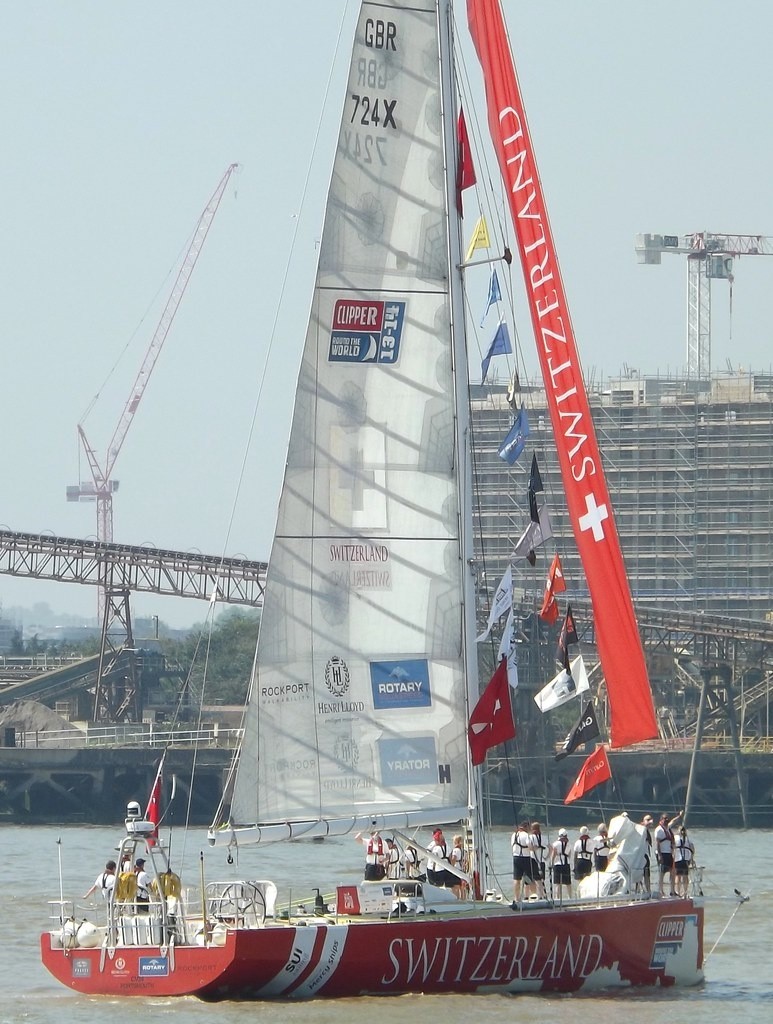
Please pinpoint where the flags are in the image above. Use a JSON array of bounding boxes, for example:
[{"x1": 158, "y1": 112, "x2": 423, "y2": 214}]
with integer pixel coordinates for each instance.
[
  {"x1": 556, "y1": 604, "x2": 578, "y2": 675},
  {"x1": 540, "y1": 552, "x2": 567, "y2": 627},
  {"x1": 555, "y1": 701, "x2": 600, "y2": 761},
  {"x1": 498, "y1": 606, "x2": 519, "y2": 689},
  {"x1": 468, "y1": 655, "x2": 516, "y2": 766},
  {"x1": 534, "y1": 655, "x2": 590, "y2": 714},
  {"x1": 146, "y1": 772, "x2": 161, "y2": 854},
  {"x1": 473, "y1": 562, "x2": 514, "y2": 644},
  {"x1": 455, "y1": 105, "x2": 553, "y2": 556},
  {"x1": 565, "y1": 745, "x2": 611, "y2": 804}
]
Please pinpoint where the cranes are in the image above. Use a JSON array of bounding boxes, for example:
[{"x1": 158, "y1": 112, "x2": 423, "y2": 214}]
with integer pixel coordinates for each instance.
[
  {"x1": 67, "y1": 161, "x2": 240, "y2": 630},
  {"x1": 634, "y1": 233, "x2": 773, "y2": 382}
]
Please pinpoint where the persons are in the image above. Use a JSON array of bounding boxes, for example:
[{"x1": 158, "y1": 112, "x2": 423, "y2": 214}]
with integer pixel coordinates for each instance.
[
  {"x1": 427, "y1": 828, "x2": 452, "y2": 889},
  {"x1": 524, "y1": 823, "x2": 553, "y2": 900},
  {"x1": 573, "y1": 823, "x2": 616, "y2": 883},
  {"x1": 549, "y1": 828, "x2": 571, "y2": 899},
  {"x1": 635, "y1": 809, "x2": 695, "y2": 897},
  {"x1": 405, "y1": 838, "x2": 425, "y2": 880},
  {"x1": 134, "y1": 858, "x2": 153, "y2": 915},
  {"x1": 449, "y1": 835, "x2": 462, "y2": 900},
  {"x1": 510, "y1": 821, "x2": 538, "y2": 902},
  {"x1": 82, "y1": 860, "x2": 117, "y2": 917},
  {"x1": 355, "y1": 832, "x2": 390, "y2": 881},
  {"x1": 385, "y1": 838, "x2": 399, "y2": 880}
]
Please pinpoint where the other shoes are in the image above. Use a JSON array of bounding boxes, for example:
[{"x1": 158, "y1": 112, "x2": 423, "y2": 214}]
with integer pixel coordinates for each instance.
[{"x1": 669, "y1": 892, "x2": 678, "y2": 896}]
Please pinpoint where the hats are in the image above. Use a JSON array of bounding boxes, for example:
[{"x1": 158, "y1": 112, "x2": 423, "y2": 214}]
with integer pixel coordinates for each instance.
[
  {"x1": 558, "y1": 828, "x2": 568, "y2": 837},
  {"x1": 531, "y1": 822, "x2": 541, "y2": 829},
  {"x1": 580, "y1": 826, "x2": 589, "y2": 835},
  {"x1": 136, "y1": 859, "x2": 146, "y2": 866},
  {"x1": 598, "y1": 823, "x2": 607, "y2": 833},
  {"x1": 106, "y1": 861, "x2": 116, "y2": 869},
  {"x1": 385, "y1": 839, "x2": 393, "y2": 842},
  {"x1": 643, "y1": 815, "x2": 654, "y2": 824}
]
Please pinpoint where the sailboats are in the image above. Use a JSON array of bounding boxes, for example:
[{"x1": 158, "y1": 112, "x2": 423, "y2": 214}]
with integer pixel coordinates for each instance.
[{"x1": 41, "y1": 0, "x2": 710, "y2": 1003}]
